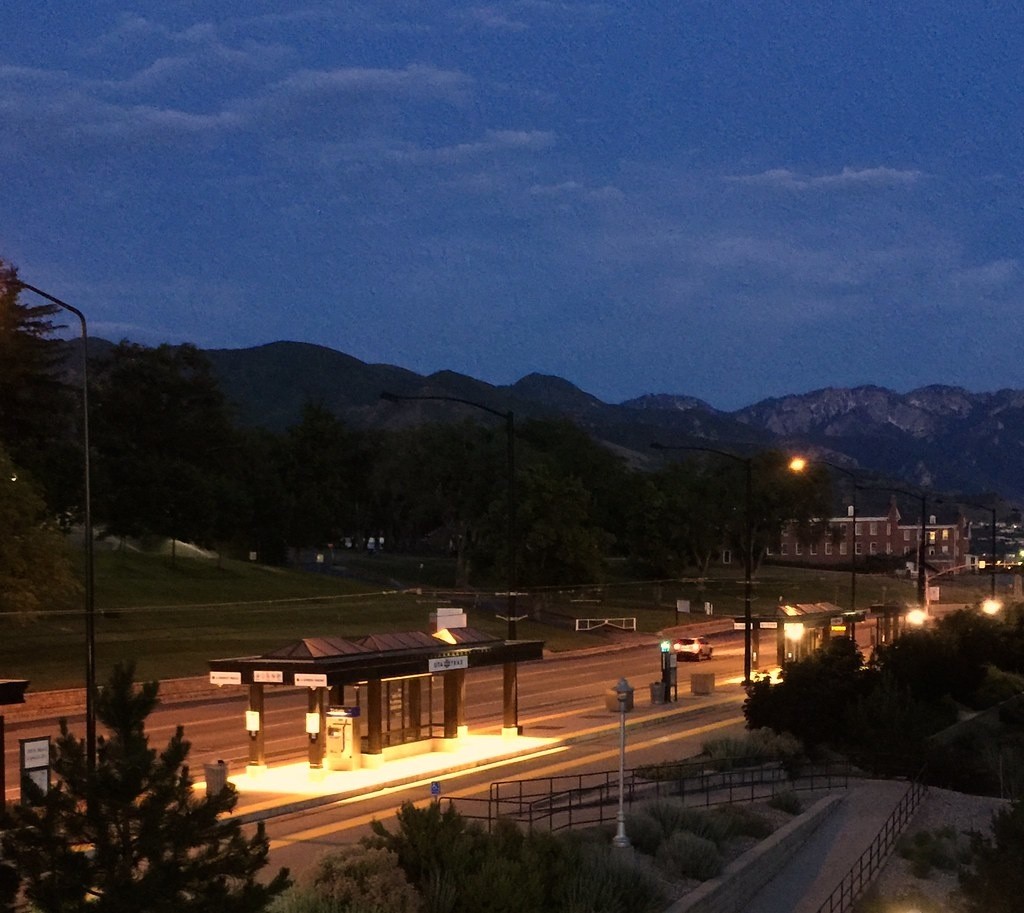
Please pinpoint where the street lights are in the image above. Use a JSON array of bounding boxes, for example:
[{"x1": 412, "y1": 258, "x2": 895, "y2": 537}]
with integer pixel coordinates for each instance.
[
  {"x1": 788, "y1": 457, "x2": 858, "y2": 645},
  {"x1": 854, "y1": 483, "x2": 927, "y2": 608},
  {"x1": 0, "y1": 278, "x2": 100, "y2": 827},
  {"x1": 379, "y1": 391, "x2": 518, "y2": 641},
  {"x1": 935, "y1": 496, "x2": 996, "y2": 601},
  {"x1": 609, "y1": 676, "x2": 636, "y2": 849},
  {"x1": 649, "y1": 440, "x2": 752, "y2": 684}
]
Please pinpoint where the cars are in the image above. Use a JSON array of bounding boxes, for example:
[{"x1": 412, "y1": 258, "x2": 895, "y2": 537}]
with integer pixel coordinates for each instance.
[{"x1": 673, "y1": 637, "x2": 714, "y2": 663}]
